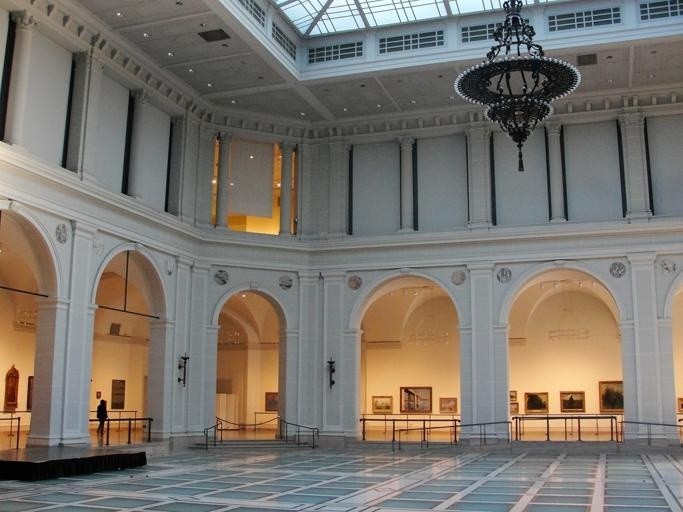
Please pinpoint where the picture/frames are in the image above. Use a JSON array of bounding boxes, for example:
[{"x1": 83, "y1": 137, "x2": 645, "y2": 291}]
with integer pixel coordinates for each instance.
[
  {"x1": 560, "y1": 390, "x2": 586, "y2": 412},
  {"x1": 372, "y1": 395, "x2": 394, "y2": 414},
  {"x1": 27, "y1": 375, "x2": 34, "y2": 411},
  {"x1": 678, "y1": 398, "x2": 683, "y2": 412},
  {"x1": 524, "y1": 392, "x2": 549, "y2": 413},
  {"x1": 400, "y1": 386, "x2": 432, "y2": 414},
  {"x1": 510, "y1": 402, "x2": 519, "y2": 414},
  {"x1": 598, "y1": 380, "x2": 624, "y2": 412},
  {"x1": 265, "y1": 392, "x2": 280, "y2": 412},
  {"x1": 440, "y1": 398, "x2": 457, "y2": 413},
  {"x1": 509, "y1": 391, "x2": 517, "y2": 401},
  {"x1": 5, "y1": 365, "x2": 19, "y2": 408}
]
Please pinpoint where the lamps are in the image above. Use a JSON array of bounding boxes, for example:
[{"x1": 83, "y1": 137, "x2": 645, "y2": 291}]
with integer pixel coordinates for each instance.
[
  {"x1": 327, "y1": 356, "x2": 335, "y2": 389},
  {"x1": 454, "y1": 0, "x2": 581, "y2": 170},
  {"x1": 177, "y1": 352, "x2": 189, "y2": 387}
]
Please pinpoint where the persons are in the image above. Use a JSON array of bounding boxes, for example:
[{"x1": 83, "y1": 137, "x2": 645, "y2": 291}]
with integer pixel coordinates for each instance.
[{"x1": 96, "y1": 400, "x2": 110, "y2": 435}]
